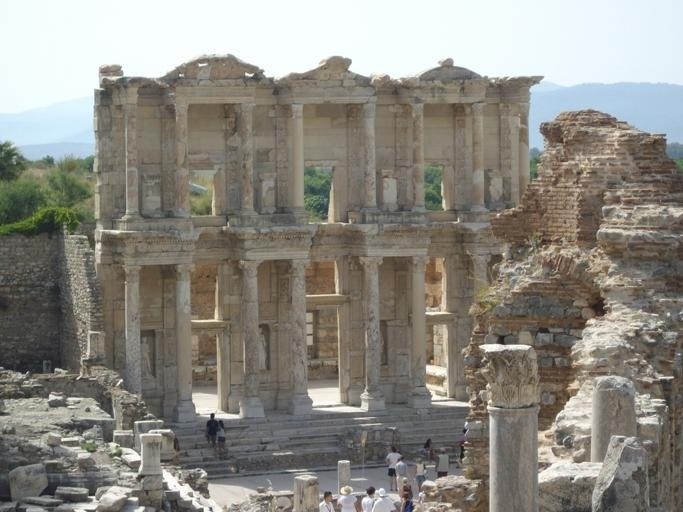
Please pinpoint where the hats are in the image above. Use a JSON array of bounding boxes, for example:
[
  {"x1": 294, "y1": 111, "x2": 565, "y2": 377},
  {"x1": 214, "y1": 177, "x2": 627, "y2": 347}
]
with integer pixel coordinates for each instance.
[
  {"x1": 379, "y1": 488, "x2": 386, "y2": 497},
  {"x1": 341, "y1": 486, "x2": 353, "y2": 495}
]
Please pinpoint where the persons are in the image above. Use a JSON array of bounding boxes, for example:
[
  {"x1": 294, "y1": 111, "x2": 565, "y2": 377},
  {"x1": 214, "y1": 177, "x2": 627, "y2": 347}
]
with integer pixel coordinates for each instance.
[
  {"x1": 360, "y1": 486, "x2": 375, "y2": 512},
  {"x1": 216, "y1": 419, "x2": 225, "y2": 453},
  {"x1": 318, "y1": 490, "x2": 335, "y2": 512},
  {"x1": 205, "y1": 413, "x2": 217, "y2": 449},
  {"x1": 336, "y1": 485, "x2": 362, "y2": 512},
  {"x1": 382, "y1": 437, "x2": 450, "y2": 511},
  {"x1": 456, "y1": 428, "x2": 467, "y2": 468},
  {"x1": 371, "y1": 488, "x2": 398, "y2": 511}
]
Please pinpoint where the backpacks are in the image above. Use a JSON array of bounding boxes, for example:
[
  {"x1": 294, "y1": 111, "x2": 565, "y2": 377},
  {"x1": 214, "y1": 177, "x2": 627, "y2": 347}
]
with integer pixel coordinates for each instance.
[{"x1": 208, "y1": 420, "x2": 217, "y2": 435}]
